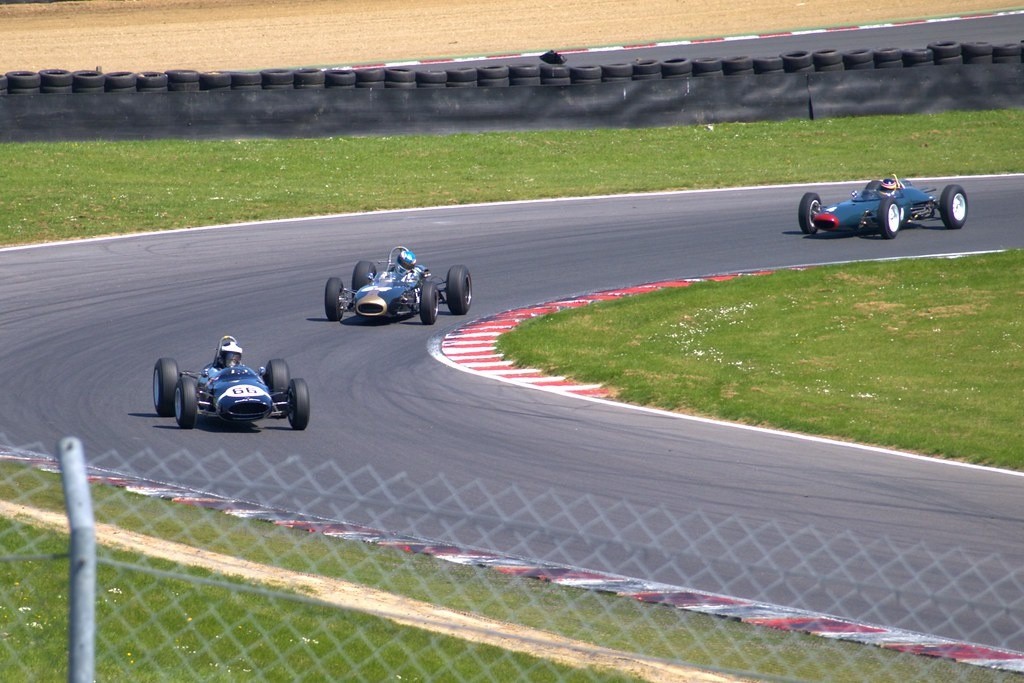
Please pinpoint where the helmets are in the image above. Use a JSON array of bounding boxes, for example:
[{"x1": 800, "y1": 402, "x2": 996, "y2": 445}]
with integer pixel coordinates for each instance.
[
  {"x1": 879, "y1": 179, "x2": 896, "y2": 196},
  {"x1": 220, "y1": 341, "x2": 243, "y2": 368},
  {"x1": 394, "y1": 250, "x2": 417, "y2": 276}
]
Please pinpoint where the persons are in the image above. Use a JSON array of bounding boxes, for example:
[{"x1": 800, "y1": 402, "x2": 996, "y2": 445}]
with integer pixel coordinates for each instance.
[
  {"x1": 880, "y1": 179, "x2": 896, "y2": 197},
  {"x1": 379, "y1": 251, "x2": 416, "y2": 281},
  {"x1": 221, "y1": 342, "x2": 242, "y2": 367}
]
